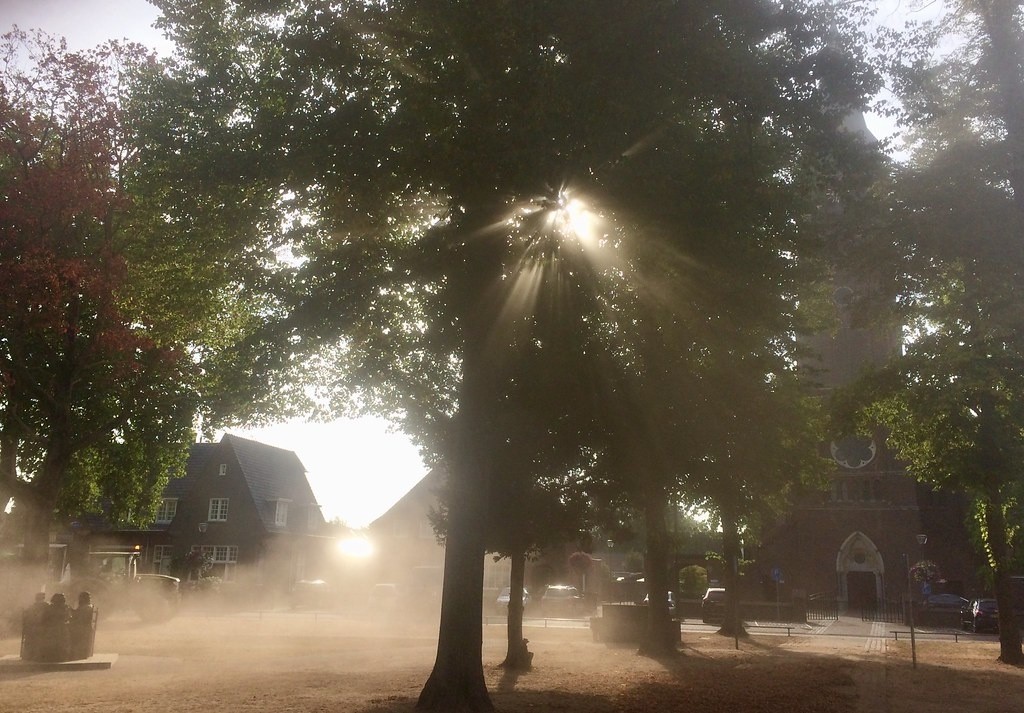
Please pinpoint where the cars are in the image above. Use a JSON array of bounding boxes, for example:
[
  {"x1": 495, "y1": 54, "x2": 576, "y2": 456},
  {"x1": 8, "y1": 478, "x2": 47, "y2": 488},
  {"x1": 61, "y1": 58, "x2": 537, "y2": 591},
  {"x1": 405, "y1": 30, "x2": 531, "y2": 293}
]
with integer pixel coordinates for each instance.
[
  {"x1": 495, "y1": 587, "x2": 532, "y2": 613},
  {"x1": 644, "y1": 591, "x2": 675, "y2": 608}
]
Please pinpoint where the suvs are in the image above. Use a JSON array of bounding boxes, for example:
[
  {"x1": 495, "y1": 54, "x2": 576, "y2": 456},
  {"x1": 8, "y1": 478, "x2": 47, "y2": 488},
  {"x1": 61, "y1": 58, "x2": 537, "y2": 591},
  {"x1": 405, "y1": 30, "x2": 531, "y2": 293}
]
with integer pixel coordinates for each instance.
[
  {"x1": 960, "y1": 595, "x2": 999, "y2": 633},
  {"x1": 541, "y1": 585, "x2": 587, "y2": 617},
  {"x1": 702, "y1": 586, "x2": 725, "y2": 623}
]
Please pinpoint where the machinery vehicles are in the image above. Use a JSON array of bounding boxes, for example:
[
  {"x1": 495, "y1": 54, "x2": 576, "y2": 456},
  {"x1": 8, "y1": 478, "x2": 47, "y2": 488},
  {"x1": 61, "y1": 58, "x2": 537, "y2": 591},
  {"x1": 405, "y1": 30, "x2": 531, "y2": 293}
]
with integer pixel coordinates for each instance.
[{"x1": 0, "y1": 539, "x2": 179, "y2": 624}]
